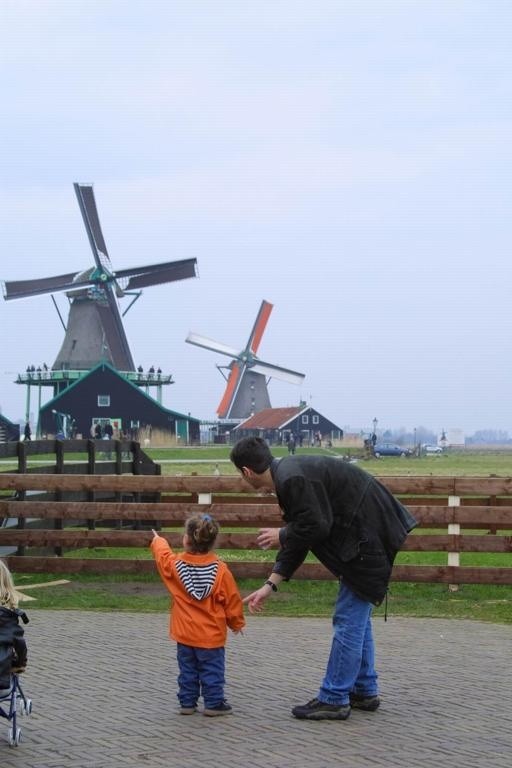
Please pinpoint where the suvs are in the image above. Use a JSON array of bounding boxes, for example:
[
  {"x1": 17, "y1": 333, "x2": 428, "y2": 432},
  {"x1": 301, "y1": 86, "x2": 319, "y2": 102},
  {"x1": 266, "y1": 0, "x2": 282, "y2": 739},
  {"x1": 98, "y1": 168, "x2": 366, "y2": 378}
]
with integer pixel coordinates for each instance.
[
  {"x1": 422, "y1": 444, "x2": 442, "y2": 454},
  {"x1": 374, "y1": 443, "x2": 409, "y2": 458}
]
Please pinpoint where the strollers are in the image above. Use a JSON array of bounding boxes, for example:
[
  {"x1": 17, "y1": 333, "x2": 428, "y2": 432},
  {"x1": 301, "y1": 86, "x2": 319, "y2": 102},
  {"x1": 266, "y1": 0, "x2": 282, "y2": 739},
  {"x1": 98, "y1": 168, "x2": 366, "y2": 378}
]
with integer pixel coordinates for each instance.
[{"x1": 1, "y1": 606, "x2": 32, "y2": 749}]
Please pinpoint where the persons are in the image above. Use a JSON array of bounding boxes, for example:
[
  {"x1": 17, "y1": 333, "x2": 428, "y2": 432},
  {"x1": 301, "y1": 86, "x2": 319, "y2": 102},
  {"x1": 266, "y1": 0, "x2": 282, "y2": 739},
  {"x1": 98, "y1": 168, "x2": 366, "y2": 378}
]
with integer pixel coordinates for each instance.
[
  {"x1": 368, "y1": 434, "x2": 372, "y2": 445},
  {"x1": 138, "y1": 365, "x2": 143, "y2": 380},
  {"x1": 0, "y1": 560, "x2": 29, "y2": 700},
  {"x1": 372, "y1": 433, "x2": 377, "y2": 445},
  {"x1": 26, "y1": 362, "x2": 48, "y2": 380},
  {"x1": 148, "y1": 365, "x2": 154, "y2": 379},
  {"x1": 90, "y1": 420, "x2": 113, "y2": 440},
  {"x1": 229, "y1": 436, "x2": 419, "y2": 719},
  {"x1": 150, "y1": 512, "x2": 246, "y2": 717},
  {"x1": 23, "y1": 422, "x2": 31, "y2": 441},
  {"x1": 288, "y1": 429, "x2": 322, "y2": 456},
  {"x1": 157, "y1": 367, "x2": 161, "y2": 380}
]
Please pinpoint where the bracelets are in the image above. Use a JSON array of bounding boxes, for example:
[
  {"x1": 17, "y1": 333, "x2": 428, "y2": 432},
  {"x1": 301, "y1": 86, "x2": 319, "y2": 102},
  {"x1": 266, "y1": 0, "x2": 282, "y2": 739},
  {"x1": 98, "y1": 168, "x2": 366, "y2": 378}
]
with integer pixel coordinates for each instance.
[{"x1": 265, "y1": 580, "x2": 278, "y2": 592}]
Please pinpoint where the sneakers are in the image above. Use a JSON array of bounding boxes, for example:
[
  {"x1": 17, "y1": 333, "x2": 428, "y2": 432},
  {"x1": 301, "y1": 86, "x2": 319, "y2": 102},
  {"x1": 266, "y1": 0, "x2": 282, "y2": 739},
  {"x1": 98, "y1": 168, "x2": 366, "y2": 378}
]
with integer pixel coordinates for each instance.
[
  {"x1": 350, "y1": 691, "x2": 380, "y2": 712},
  {"x1": 180, "y1": 703, "x2": 199, "y2": 715},
  {"x1": 203, "y1": 699, "x2": 234, "y2": 717},
  {"x1": 292, "y1": 697, "x2": 352, "y2": 720}
]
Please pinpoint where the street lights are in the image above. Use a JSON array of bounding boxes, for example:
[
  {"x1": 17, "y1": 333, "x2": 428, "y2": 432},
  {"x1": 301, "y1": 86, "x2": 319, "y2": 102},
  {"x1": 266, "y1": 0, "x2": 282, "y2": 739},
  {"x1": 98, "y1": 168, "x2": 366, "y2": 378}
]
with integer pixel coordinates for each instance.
[{"x1": 373, "y1": 418, "x2": 378, "y2": 433}]
